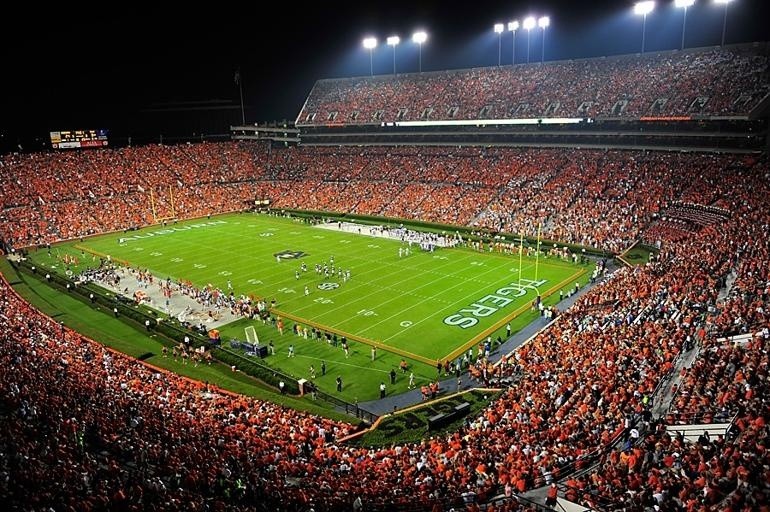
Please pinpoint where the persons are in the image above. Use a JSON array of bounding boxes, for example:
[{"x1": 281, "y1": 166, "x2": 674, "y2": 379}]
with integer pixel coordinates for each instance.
[
  {"x1": 490, "y1": 250, "x2": 767, "y2": 511},
  {"x1": 298, "y1": 39, "x2": 769, "y2": 127},
  {"x1": 463, "y1": 336, "x2": 492, "y2": 366},
  {"x1": 287, "y1": 324, "x2": 351, "y2": 360},
  {"x1": 1, "y1": 139, "x2": 769, "y2": 256},
  {"x1": 296, "y1": 255, "x2": 351, "y2": 296},
  {"x1": 45, "y1": 250, "x2": 283, "y2": 368},
  {"x1": 107, "y1": 356, "x2": 491, "y2": 511},
  {"x1": 0, "y1": 276, "x2": 113, "y2": 512}
]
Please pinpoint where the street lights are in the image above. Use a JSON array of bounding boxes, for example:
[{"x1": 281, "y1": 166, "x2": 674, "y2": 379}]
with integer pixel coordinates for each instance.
[
  {"x1": 676, "y1": 0, "x2": 695, "y2": 49},
  {"x1": 714, "y1": 0, "x2": 733, "y2": 46},
  {"x1": 494, "y1": 16, "x2": 550, "y2": 65},
  {"x1": 363, "y1": 38, "x2": 377, "y2": 76},
  {"x1": 634, "y1": 0, "x2": 655, "y2": 51},
  {"x1": 412, "y1": 31, "x2": 427, "y2": 72},
  {"x1": 387, "y1": 35, "x2": 399, "y2": 74}
]
